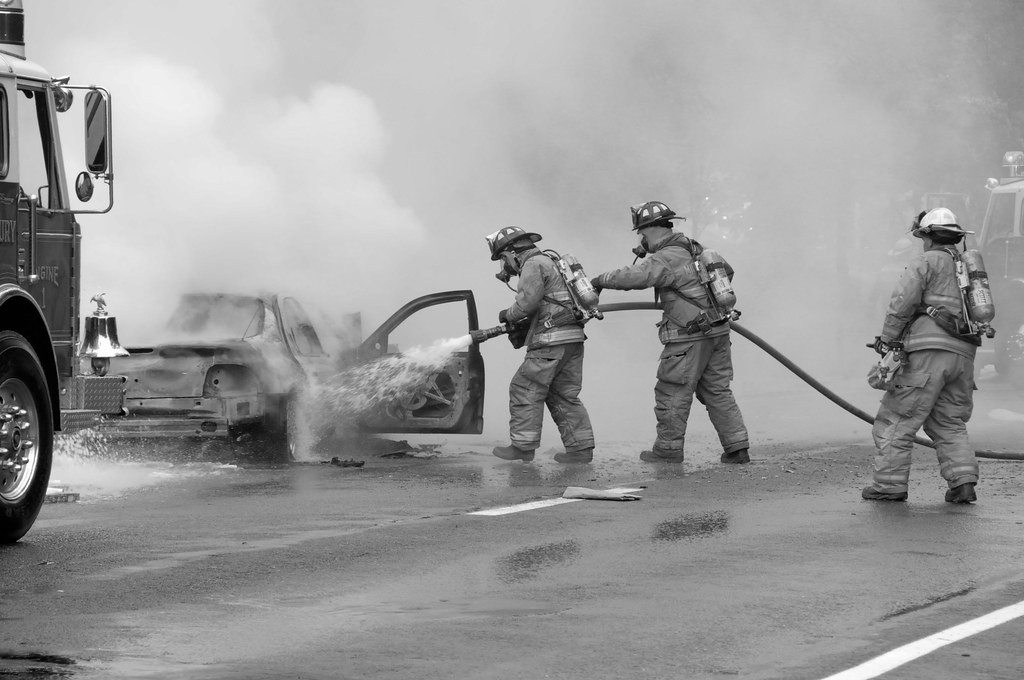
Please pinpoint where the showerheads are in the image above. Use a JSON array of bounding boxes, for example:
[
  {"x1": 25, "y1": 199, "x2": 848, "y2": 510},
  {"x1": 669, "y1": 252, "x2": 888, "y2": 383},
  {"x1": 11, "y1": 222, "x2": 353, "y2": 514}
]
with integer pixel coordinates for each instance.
[{"x1": 469, "y1": 326, "x2": 502, "y2": 345}]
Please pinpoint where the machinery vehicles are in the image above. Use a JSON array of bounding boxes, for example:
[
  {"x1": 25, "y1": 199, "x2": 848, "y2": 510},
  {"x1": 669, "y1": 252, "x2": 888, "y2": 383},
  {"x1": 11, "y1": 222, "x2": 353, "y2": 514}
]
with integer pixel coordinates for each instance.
[{"x1": 0, "y1": 0, "x2": 130, "y2": 543}]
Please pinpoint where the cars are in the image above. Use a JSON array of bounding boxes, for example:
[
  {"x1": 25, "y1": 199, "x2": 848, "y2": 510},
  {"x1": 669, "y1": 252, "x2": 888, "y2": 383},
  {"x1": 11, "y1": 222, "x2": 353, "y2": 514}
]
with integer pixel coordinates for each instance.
[
  {"x1": 79, "y1": 290, "x2": 485, "y2": 448},
  {"x1": 918, "y1": 151, "x2": 1023, "y2": 386}
]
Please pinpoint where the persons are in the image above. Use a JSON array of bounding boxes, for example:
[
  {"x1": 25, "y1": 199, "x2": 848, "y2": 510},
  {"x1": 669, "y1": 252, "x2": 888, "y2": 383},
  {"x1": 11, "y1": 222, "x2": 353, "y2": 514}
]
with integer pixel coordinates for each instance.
[
  {"x1": 590, "y1": 201, "x2": 750, "y2": 464},
  {"x1": 484, "y1": 227, "x2": 596, "y2": 464},
  {"x1": 861, "y1": 207, "x2": 981, "y2": 504}
]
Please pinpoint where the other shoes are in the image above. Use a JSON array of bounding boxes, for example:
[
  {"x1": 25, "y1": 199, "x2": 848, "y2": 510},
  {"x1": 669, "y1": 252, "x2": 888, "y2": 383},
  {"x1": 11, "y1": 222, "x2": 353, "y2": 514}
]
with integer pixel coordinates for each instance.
[
  {"x1": 492, "y1": 444, "x2": 535, "y2": 461},
  {"x1": 640, "y1": 450, "x2": 684, "y2": 463},
  {"x1": 861, "y1": 486, "x2": 908, "y2": 500},
  {"x1": 945, "y1": 482, "x2": 977, "y2": 503},
  {"x1": 721, "y1": 449, "x2": 750, "y2": 464},
  {"x1": 554, "y1": 449, "x2": 593, "y2": 464}
]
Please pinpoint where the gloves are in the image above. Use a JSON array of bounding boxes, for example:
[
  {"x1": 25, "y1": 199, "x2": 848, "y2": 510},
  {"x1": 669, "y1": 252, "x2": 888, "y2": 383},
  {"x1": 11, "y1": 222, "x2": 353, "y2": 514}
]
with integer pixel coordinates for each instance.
[
  {"x1": 499, "y1": 309, "x2": 507, "y2": 323},
  {"x1": 591, "y1": 275, "x2": 604, "y2": 295},
  {"x1": 508, "y1": 331, "x2": 525, "y2": 349},
  {"x1": 874, "y1": 340, "x2": 883, "y2": 355}
]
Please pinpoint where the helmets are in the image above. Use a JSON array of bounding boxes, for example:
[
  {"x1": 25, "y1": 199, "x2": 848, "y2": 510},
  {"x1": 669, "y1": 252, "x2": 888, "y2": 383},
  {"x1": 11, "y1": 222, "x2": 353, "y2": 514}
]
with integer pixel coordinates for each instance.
[
  {"x1": 491, "y1": 226, "x2": 543, "y2": 260},
  {"x1": 912, "y1": 208, "x2": 975, "y2": 237},
  {"x1": 631, "y1": 201, "x2": 675, "y2": 230}
]
